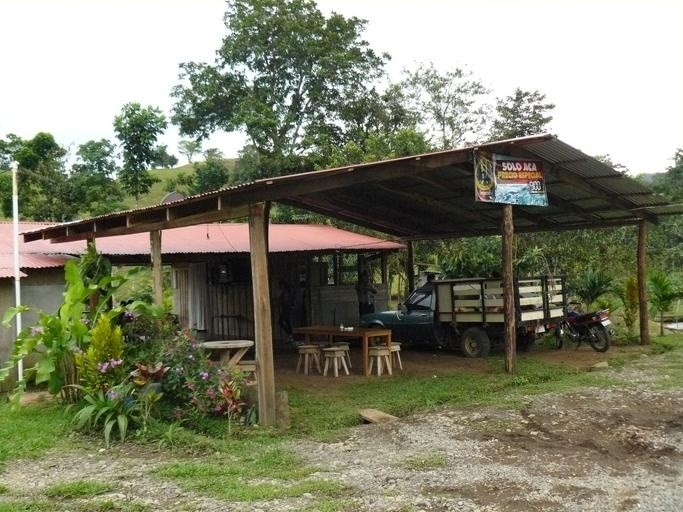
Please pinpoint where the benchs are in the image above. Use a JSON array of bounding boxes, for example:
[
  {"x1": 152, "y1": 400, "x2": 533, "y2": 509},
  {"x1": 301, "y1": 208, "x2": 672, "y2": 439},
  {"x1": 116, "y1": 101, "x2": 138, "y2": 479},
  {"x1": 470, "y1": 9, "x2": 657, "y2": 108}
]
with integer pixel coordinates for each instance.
[
  {"x1": 215, "y1": 365, "x2": 255, "y2": 380},
  {"x1": 208, "y1": 360, "x2": 255, "y2": 365}
]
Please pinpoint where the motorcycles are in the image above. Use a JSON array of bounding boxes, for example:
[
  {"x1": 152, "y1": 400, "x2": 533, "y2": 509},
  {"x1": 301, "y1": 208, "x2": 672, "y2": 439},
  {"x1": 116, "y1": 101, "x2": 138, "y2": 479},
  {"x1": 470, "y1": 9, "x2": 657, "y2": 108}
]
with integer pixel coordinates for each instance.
[{"x1": 554, "y1": 300, "x2": 614, "y2": 353}]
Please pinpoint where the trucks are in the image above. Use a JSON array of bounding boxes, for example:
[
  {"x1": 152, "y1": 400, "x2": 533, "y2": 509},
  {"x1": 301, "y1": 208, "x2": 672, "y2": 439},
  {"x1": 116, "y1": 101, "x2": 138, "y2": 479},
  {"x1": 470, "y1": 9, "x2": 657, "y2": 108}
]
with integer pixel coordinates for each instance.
[{"x1": 360, "y1": 275, "x2": 568, "y2": 359}]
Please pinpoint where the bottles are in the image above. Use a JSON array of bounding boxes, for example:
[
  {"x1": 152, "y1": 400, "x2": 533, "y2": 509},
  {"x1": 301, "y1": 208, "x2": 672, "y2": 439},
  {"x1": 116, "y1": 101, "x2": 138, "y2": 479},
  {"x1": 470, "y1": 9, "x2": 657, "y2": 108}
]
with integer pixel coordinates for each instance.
[{"x1": 338, "y1": 323, "x2": 343, "y2": 332}]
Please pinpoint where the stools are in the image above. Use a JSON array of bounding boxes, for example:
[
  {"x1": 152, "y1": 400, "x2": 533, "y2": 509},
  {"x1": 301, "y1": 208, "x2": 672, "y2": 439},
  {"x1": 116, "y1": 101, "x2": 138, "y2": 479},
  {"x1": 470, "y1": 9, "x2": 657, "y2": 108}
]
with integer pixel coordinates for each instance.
[{"x1": 294, "y1": 342, "x2": 404, "y2": 379}]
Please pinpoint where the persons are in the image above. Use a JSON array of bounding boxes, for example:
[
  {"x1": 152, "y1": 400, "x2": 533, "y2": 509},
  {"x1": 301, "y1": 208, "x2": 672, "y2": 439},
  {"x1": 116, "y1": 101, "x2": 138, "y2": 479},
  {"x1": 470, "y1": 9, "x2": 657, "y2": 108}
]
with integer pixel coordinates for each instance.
[
  {"x1": 355, "y1": 272, "x2": 378, "y2": 345},
  {"x1": 278, "y1": 279, "x2": 295, "y2": 344},
  {"x1": 426, "y1": 273, "x2": 435, "y2": 283}
]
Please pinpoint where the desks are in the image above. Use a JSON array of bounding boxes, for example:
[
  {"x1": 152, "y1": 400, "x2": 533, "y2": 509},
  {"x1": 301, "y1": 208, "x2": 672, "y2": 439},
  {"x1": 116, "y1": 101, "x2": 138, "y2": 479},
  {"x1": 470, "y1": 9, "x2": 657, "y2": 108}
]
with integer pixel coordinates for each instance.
[
  {"x1": 191, "y1": 340, "x2": 254, "y2": 368},
  {"x1": 290, "y1": 324, "x2": 392, "y2": 377}
]
